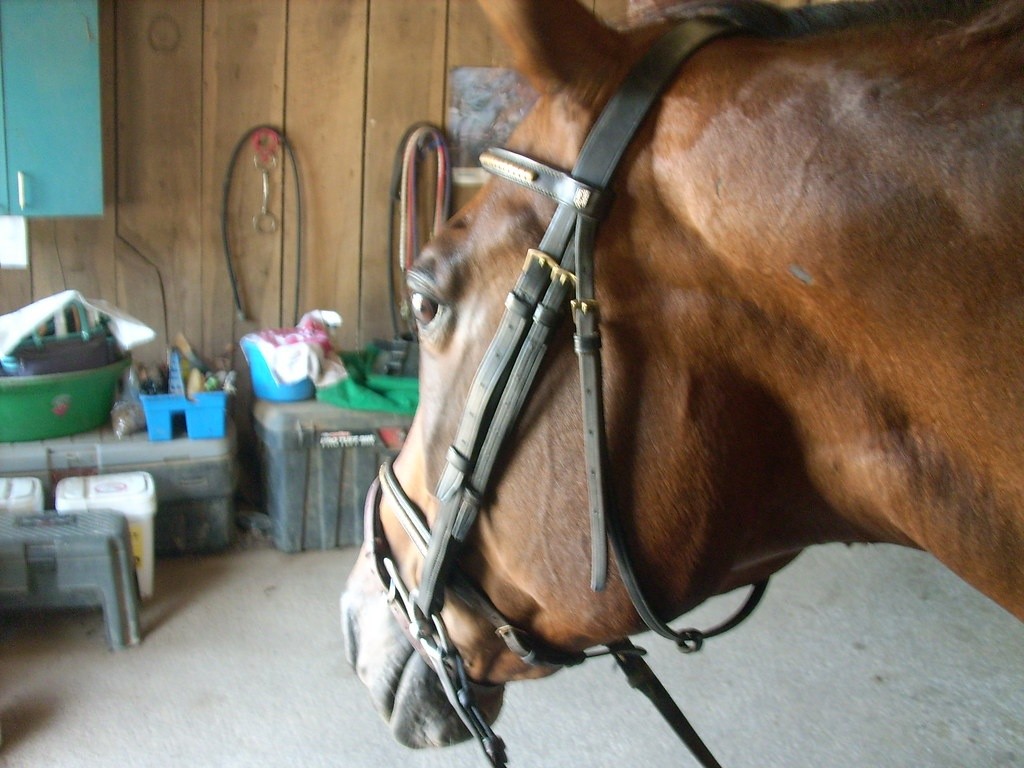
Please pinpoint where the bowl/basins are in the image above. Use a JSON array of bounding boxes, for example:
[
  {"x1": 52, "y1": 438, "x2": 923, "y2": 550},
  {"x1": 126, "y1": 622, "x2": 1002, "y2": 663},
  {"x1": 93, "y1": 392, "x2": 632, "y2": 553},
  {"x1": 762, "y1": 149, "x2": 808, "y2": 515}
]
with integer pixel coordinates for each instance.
[{"x1": 0, "y1": 354, "x2": 132, "y2": 443}]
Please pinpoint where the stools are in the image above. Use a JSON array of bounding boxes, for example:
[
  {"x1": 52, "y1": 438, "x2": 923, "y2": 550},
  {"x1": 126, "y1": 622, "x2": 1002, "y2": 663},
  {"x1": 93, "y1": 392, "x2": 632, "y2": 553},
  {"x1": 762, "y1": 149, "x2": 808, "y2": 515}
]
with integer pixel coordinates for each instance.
[{"x1": 0, "y1": 509, "x2": 143, "y2": 654}]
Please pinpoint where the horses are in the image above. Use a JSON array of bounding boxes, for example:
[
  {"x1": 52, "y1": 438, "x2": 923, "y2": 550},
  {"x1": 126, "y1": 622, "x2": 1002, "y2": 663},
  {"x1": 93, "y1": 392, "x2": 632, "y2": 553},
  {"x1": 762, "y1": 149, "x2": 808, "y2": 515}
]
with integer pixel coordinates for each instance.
[{"x1": 342, "y1": 2, "x2": 1024, "y2": 752}]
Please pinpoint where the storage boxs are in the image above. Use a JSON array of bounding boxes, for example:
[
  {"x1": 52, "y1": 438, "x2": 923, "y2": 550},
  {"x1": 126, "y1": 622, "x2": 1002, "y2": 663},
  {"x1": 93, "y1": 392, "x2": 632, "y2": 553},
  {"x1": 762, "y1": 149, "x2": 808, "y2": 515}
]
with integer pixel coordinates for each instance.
[
  {"x1": 252, "y1": 402, "x2": 413, "y2": 552},
  {"x1": 54, "y1": 470, "x2": 159, "y2": 600},
  {"x1": 0, "y1": 477, "x2": 45, "y2": 513},
  {"x1": 0, "y1": 413, "x2": 244, "y2": 556}
]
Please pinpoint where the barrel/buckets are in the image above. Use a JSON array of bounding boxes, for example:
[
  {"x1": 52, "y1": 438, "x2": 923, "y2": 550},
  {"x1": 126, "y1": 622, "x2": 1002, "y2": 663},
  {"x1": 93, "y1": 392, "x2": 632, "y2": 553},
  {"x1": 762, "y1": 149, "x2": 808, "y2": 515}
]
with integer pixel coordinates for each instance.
[
  {"x1": 242, "y1": 337, "x2": 315, "y2": 403},
  {"x1": 0, "y1": 477, "x2": 43, "y2": 513},
  {"x1": 55, "y1": 471, "x2": 156, "y2": 600}
]
